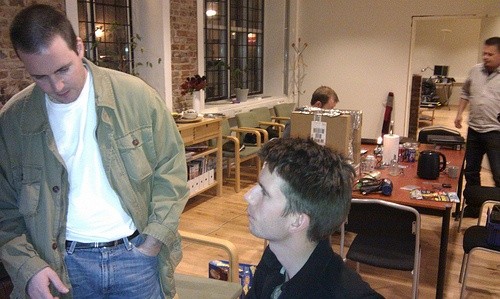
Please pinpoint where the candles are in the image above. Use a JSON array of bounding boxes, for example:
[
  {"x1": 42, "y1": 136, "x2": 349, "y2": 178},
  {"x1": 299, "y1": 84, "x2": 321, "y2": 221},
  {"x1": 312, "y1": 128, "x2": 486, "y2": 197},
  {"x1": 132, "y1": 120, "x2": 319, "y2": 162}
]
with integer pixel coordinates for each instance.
[{"x1": 382, "y1": 130, "x2": 399, "y2": 164}]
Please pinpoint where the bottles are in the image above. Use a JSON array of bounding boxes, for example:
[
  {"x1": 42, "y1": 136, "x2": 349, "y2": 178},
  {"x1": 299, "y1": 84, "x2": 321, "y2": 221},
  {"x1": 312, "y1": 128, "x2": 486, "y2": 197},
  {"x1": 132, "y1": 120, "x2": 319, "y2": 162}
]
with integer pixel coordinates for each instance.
[
  {"x1": 373, "y1": 137, "x2": 385, "y2": 169},
  {"x1": 388, "y1": 159, "x2": 400, "y2": 176}
]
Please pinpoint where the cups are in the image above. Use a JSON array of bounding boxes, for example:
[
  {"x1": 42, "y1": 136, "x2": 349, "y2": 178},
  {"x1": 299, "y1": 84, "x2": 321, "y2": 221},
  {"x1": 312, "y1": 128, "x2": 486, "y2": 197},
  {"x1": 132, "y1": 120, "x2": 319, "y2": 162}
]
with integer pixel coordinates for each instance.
[{"x1": 448, "y1": 166, "x2": 459, "y2": 178}]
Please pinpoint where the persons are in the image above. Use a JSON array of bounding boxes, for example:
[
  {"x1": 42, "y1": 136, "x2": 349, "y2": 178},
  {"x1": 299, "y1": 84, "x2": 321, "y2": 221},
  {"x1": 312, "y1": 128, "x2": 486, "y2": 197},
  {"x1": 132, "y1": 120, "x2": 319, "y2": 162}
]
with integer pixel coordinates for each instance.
[
  {"x1": 282, "y1": 86, "x2": 340, "y2": 137},
  {"x1": 0, "y1": 4, "x2": 189, "y2": 299},
  {"x1": 243, "y1": 138, "x2": 386, "y2": 299},
  {"x1": 453, "y1": 36, "x2": 500, "y2": 218}
]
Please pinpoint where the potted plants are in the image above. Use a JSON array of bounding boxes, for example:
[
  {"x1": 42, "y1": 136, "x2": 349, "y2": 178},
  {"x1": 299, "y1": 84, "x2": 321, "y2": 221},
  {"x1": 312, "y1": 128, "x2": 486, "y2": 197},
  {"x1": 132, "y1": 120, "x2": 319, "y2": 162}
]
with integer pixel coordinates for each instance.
[{"x1": 232, "y1": 68, "x2": 249, "y2": 102}]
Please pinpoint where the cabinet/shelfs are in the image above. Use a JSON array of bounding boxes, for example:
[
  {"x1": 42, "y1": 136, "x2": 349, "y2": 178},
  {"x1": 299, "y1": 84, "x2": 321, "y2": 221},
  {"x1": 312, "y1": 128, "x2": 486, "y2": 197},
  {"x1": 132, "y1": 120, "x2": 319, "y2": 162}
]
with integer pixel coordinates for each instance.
[{"x1": 419, "y1": 82, "x2": 465, "y2": 110}]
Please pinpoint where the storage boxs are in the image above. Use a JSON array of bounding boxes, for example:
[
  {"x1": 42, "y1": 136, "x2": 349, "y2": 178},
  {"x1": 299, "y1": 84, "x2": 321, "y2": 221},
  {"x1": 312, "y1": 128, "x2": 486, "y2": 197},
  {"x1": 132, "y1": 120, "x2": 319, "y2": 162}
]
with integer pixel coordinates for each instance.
[
  {"x1": 292, "y1": 107, "x2": 363, "y2": 164},
  {"x1": 187, "y1": 168, "x2": 215, "y2": 197},
  {"x1": 426, "y1": 134, "x2": 466, "y2": 150}
]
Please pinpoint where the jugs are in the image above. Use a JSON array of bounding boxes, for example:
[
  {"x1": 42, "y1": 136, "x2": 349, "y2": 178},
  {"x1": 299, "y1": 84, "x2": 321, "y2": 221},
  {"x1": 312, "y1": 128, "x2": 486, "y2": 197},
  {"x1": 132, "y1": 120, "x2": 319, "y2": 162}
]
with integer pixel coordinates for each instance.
[{"x1": 417, "y1": 149, "x2": 446, "y2": 180}]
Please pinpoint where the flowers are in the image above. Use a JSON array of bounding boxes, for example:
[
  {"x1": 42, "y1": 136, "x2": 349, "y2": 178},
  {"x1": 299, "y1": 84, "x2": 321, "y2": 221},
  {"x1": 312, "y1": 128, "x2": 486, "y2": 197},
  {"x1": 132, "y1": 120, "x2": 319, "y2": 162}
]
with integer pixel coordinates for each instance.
[{"x1": 180, "y1": 73, "x2": 207, "y2": 96}]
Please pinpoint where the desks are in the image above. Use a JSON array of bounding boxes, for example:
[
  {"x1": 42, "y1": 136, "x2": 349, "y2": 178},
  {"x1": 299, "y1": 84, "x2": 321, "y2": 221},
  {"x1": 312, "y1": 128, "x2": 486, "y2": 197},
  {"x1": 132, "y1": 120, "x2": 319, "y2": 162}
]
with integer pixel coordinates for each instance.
[
  {"x1": 351, "y1": 144, "x2": 466, "y2": 299},
  {"x1": 176, "y1": 118, "x2": 224, "y2": 199}
]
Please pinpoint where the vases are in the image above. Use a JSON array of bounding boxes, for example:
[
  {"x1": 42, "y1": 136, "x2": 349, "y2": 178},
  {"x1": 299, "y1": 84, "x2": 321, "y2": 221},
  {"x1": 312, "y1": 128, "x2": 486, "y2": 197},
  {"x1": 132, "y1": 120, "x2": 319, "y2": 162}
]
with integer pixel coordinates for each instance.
[{"x1": 192, "y1": 90, "x2": 206, "y2": 116}]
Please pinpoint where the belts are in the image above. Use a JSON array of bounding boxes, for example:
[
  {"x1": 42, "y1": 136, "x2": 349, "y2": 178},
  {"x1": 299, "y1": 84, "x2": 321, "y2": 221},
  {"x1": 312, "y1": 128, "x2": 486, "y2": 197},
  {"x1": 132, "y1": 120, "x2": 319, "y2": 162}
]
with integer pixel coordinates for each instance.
[{"x1": 65, "y1": 229, "x2": 139, "y2": 248}]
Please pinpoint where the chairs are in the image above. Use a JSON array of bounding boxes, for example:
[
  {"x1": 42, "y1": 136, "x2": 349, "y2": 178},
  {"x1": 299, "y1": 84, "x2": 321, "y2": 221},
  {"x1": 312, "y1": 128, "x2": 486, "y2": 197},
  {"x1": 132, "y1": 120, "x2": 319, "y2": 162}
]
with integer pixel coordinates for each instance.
[
  {"x1": 417, "y1": 126, "x2": 460, "y2": 145},
  {"x1": 340, "y1": 198, "x2": 421, "y2": 299},
  {"x1": 170, "y1": 230, "x2": 243, "y2": 299},
  {"x1": 457, "y1": 186, "x2": 500, "y2": 299},
  {"x1": 205, "y1": 103, "x2": 291, "y2": 193}
]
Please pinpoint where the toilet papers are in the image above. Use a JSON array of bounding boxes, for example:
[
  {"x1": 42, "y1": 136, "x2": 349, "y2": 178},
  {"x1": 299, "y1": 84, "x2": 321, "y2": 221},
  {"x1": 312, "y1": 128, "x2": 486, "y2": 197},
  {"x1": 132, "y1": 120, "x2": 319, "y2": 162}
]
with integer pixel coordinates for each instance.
[{"x1": 382, "y1": 133, "x2": 400, "y2": 166}]
